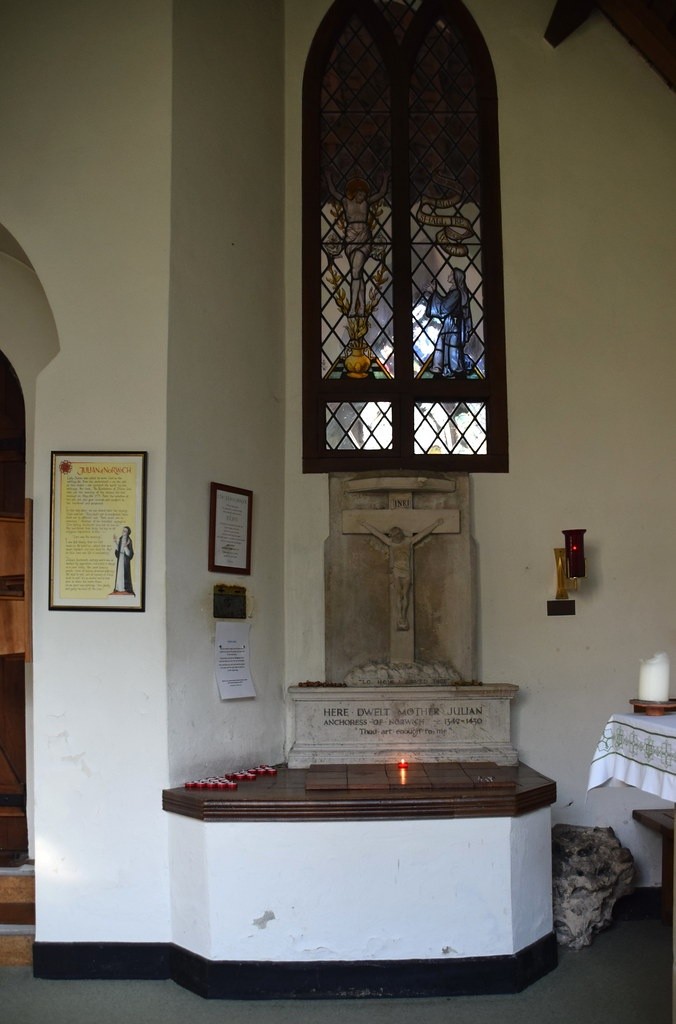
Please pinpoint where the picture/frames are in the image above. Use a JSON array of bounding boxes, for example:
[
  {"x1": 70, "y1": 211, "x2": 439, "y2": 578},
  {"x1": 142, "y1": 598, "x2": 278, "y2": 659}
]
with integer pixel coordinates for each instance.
[{"x1": 49, "y1": 450, "x2": 145, "y2": 611}]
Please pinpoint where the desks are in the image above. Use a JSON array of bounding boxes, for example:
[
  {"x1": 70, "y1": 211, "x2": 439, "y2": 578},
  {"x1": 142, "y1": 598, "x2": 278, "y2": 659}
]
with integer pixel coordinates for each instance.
[{"x1": 588, "y1": 713, "x2": 676, "y2": 802}]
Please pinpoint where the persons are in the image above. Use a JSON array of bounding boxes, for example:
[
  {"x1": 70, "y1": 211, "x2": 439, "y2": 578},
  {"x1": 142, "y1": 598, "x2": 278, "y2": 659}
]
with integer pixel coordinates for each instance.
[{"x1": 358, "y1": 518, "x2": 444, "y2": 629}]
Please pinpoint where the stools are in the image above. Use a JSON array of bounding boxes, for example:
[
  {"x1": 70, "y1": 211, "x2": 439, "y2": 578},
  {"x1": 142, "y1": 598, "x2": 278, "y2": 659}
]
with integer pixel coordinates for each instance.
[{"x1": 632, "y1": 809, "x2": 673, "y2": 924}]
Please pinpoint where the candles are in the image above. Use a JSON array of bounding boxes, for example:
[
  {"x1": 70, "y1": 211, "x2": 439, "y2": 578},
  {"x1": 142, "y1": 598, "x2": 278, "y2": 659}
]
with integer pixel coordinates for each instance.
[
  {"x1": 638, "y1": 653, "x2": 668, "y2": 700},
  {"x1": 187, "y1": 764, "x2": 277, "y2": 790},
  {"x1": 398, "y1": 762, "x2": 408, "y2": 767}
]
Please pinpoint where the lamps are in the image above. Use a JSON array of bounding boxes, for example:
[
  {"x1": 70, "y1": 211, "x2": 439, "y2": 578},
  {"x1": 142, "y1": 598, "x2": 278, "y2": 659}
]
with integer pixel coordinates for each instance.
[{"x1": 554, "y1": 527, "x2": 586, "y2": 600}]
